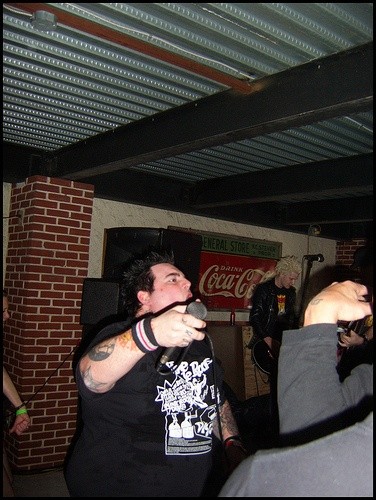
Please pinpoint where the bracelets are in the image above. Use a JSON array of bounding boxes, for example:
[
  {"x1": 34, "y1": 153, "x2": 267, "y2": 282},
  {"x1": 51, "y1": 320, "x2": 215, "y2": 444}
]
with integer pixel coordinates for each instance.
[
  {"x1": 15, "y1": 409, "x2": 27, "y2": 416},
  {"x1": 131, "y1": 315, "x2": 161, "y2": 353},
  {"x1": 221, "y1": 433, "x2": 244, "y2": 446}
]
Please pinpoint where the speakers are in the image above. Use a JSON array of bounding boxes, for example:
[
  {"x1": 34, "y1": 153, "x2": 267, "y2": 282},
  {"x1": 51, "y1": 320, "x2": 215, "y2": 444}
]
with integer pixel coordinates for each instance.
[{"x1": 80, "y1": 280, "x2": 129, "y2": 326}]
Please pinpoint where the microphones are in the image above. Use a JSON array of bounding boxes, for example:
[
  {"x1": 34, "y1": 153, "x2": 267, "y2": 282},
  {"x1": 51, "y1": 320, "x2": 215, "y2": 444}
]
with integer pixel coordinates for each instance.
[
  {"x1": 304, "y1": 254, "x2": 324, "y2": 262},
  {"x1": 157, "y1": 302, "x2": 207, "y2": 371}
]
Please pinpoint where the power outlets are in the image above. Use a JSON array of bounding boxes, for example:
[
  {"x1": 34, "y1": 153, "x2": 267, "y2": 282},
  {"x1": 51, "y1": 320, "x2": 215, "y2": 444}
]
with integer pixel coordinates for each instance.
[{"x1": 18, "y1": 210, "x2": 25, "y2": 226}]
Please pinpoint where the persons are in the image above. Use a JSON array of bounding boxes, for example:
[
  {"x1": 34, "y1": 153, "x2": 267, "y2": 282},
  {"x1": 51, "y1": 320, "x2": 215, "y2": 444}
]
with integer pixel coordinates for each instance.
[
  {"x1": 62, "y1": 251, "x2": 247, "y2": 499},
  {"x1": 218, "y1": 409, "x2": 373, "y2": 498},
  {"x1": 2, "y1": 291, "x2": 30, "y2": 498},
  {"x1": 252, "y1": 255, "x2": 307, "y2": 391},
  {"x1": 279, "y1": 279, "x2": 375, "y2": 437}
]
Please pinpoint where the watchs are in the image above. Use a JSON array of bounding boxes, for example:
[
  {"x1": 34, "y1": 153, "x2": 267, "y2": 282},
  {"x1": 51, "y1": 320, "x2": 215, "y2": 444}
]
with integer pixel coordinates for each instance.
[{"x1": 358, "y1": 333, "x2": 370, "y2": 348}]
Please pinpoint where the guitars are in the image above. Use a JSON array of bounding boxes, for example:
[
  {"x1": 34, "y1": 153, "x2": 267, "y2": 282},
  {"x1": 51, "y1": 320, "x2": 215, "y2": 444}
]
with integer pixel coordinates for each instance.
[{"x1": 252, "y1": 340, "x2": 275, "y2": 374}]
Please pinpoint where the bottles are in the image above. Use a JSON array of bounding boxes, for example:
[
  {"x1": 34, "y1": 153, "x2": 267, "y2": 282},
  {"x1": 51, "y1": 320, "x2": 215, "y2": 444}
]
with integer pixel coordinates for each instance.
[{"x1": 230, "y1": 307, "x2": 235, "y2": 326}]
